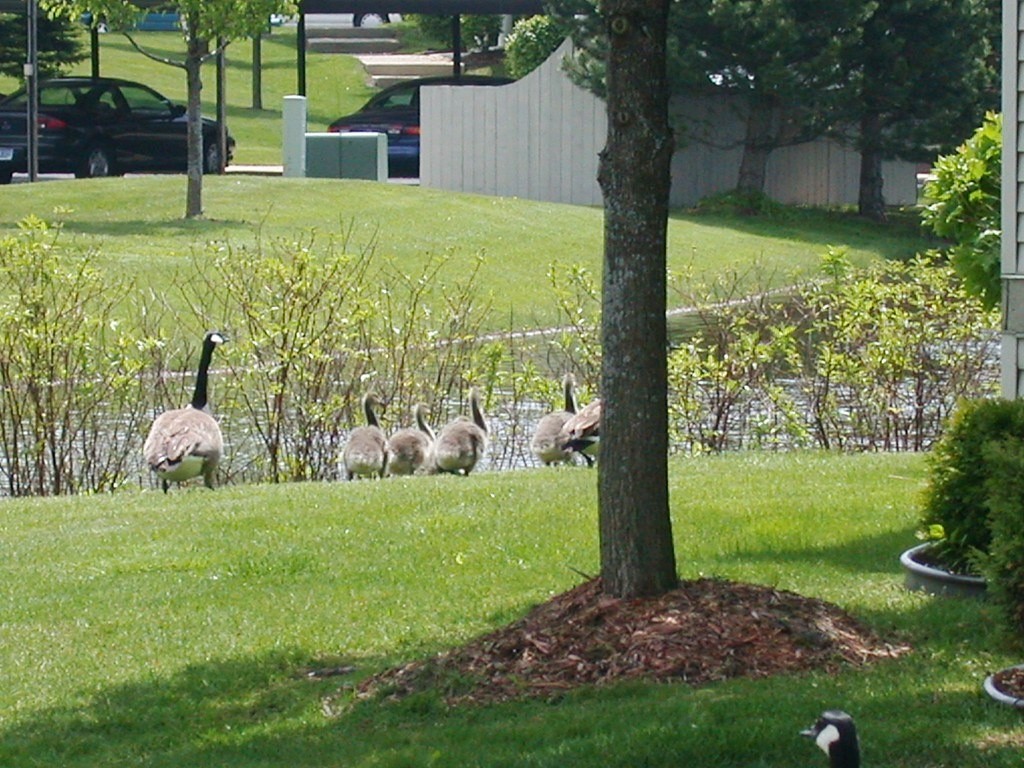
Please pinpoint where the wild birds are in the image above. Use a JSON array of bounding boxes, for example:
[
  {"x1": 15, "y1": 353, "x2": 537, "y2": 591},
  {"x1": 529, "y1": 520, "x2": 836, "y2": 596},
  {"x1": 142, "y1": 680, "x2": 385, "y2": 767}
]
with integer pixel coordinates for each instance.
[
  {"x1": 143, "y1": 328, "x2": 231, "y2": 496},
  {"x1": 529, "y1": 371, "x2": 602, "y2": 469},
  {"x1": 340, "y1": 385, "x2": 490, "y2": 482},
  {"x1": 798, "y1": 709, "x2": 862, "y2": 768}
]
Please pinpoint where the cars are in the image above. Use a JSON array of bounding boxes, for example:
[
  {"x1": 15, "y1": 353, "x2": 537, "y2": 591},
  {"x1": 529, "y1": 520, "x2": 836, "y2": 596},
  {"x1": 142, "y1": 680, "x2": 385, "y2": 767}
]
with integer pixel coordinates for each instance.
[
  {"x1": 0, "y1": 76, "x2": 235, "y2": 184},
  {"x1": 326, "y1": 74, "x2": 517, "y2": 177}
]
